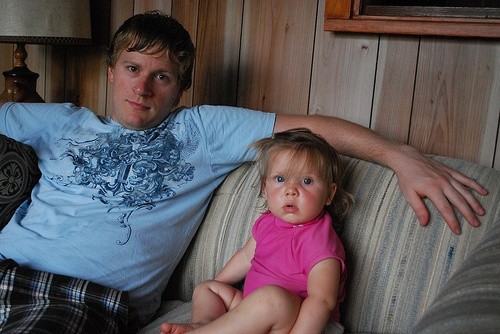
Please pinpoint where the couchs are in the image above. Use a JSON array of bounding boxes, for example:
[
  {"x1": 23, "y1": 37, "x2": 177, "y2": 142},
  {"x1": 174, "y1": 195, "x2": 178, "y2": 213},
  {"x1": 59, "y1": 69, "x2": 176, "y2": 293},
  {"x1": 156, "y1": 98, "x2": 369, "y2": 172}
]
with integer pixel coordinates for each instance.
[{"x1": 130, "y1": 147, "x2": 499, "y2": 334}]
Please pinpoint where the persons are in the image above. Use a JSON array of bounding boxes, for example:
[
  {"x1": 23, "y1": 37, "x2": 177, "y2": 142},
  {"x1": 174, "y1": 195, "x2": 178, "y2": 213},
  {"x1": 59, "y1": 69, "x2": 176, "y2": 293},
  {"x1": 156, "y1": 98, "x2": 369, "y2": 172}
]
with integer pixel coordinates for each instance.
[
  {"x1": 0, "y1": 9, "x2": 491, "y2": 334},
  {"x1": 156, "y1": 126, "x2": 353, "y2": 334}
]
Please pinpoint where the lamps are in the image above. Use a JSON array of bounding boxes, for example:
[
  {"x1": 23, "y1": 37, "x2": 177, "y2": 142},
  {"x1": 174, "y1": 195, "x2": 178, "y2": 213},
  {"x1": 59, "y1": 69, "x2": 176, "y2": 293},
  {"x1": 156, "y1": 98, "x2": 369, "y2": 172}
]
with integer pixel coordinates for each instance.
[{"x1": 0, "y1": 1, "x2": 93, "y2": 229}]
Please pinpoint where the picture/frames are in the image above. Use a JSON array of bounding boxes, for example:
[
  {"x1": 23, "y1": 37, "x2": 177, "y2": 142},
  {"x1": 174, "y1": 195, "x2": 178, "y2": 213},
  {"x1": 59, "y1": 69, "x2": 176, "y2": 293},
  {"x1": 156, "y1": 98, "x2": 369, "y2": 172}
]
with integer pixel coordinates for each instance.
[{"x1": 322, "y1": 0, "x2": 499, "y2": 40}]
